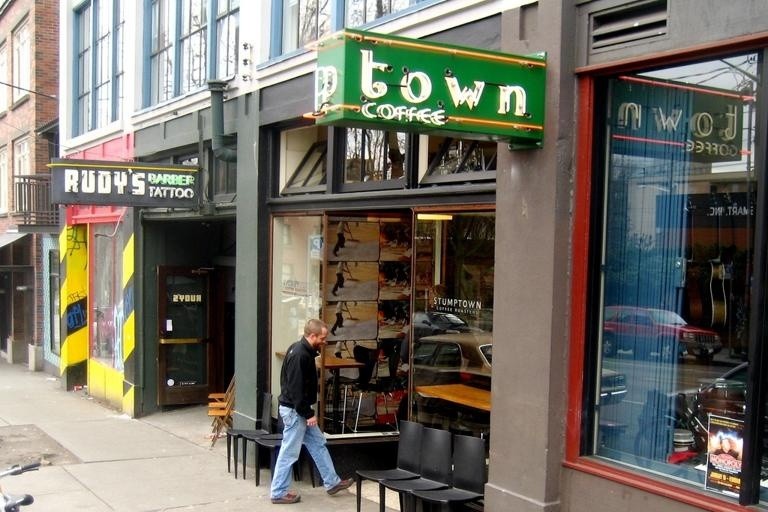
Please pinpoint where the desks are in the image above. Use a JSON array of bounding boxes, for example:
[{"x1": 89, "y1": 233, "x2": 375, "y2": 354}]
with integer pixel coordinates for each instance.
[
  {"x1": 276, "y1": 351, "x2": 362, "y2": 424},
  {"x1": 416, "y1": 384, "x2": 491, "y2": 412}
]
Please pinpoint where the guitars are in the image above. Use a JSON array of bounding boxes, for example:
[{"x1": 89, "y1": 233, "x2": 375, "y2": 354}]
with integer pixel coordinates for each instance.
[
  {"x1": 679, "y1": 192, "x2": 703, "y2": 325},
  {"x1": 709, "y1": 182, "x2": 730, "y2": 334}
]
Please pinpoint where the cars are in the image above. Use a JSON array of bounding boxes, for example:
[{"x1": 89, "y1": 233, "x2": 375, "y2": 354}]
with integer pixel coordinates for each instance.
[
  {"x1": 640, "y1": 360, "x2": 767, "y2": 451},
  {"x1": 413, "y1": 336, "x2": 627, "y2": 419}
]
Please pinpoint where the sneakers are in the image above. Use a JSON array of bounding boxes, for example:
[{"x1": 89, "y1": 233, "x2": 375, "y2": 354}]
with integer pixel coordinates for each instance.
[
  {"x1": 271, "y1": 494, "x2": 301, "y2": 504},
  {"x1": 327, "y1": 478, "x2": 354, "y2": 495}
]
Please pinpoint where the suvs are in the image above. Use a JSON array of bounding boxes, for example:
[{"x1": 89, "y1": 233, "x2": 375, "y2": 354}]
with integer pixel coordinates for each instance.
[{"x1": 602, "y1": 305, "x2": 724, "y2": 357}]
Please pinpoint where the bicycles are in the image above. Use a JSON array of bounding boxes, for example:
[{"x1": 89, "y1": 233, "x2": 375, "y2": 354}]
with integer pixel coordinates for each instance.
[{"x1": 634, "y1": 378, "x2": 768, "y2": 479}]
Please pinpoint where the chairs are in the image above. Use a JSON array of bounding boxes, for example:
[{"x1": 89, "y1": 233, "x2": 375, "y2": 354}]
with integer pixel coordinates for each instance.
[
  {"x1": 411, "y1": 434, "x2": 485, "y2": 512},
  {"x1": 207, "y1": 373, "x2": 235, "y2": 426},
  {"x1": 254, "y1": 437, "x2": 302, "y2": 487},
  {"x1": 209, "y1": 395, "x2": 234, "y2": 447},
  {"x1": 226, "y1": 392, "x2": 273, "y2": 472},
  {"x1": 240, "y1": 403, "x2": 285, "y2": 476},
  {"x1": 355, "y1": 421, "x2": 423, "y2": 512},
  {"x1": 378, "y1": 429, "x2": 452, "y2": 512},
  {"x1": 208, "y1": 382, "x2": 235, "y2": 433}
]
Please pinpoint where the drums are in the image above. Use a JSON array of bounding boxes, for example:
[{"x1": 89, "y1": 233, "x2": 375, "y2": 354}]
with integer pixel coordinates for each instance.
[{"x1": 695, "y1": 376, "x2": 746, "y2": 454}]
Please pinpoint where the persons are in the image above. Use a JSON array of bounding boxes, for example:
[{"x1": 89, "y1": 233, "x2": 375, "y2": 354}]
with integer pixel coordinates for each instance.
[
  {"x1": 331, "y1": 301, "x2": 350, "y2": 335},
  {"x1": 331, "y1": 262, "x2": 348, "y2": 297},
  {"x1": 271, "y1": 318, "x2": 354, "y2": 503},
  {"x1": 333, "y1": 222, "x2": 351, "y2": 257}
]
